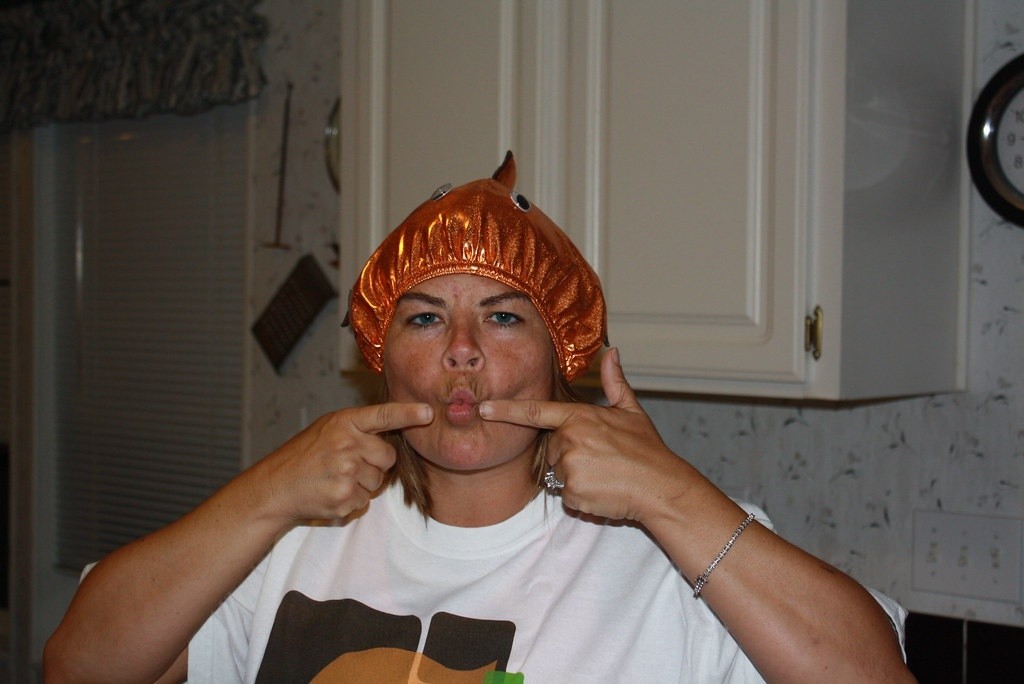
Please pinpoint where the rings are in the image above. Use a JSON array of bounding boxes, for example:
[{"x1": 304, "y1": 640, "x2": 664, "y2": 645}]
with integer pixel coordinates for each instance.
[{"x1": 543, "y1": 466, "x2": 565, "y2": 490}]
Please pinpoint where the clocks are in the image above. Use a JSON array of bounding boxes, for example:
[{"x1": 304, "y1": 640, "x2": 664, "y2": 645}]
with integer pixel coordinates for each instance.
[{"x1": 966, "y1": 50, "x2": 1024, "y2": 230}]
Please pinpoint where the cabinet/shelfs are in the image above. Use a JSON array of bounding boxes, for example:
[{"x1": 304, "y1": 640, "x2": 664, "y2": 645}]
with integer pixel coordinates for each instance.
[{"x1": 340, "y1": 0, "x2": 964, "y2": 404}]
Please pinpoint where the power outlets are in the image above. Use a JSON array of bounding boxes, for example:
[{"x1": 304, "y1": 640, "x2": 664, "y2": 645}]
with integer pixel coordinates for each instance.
[{"x1": 910, "y1": 508, "x2": 1023, "y2": 602}]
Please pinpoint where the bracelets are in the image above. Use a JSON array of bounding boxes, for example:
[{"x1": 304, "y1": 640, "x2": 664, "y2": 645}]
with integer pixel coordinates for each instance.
[{"x1": 693, "y1": 513, "x2": 756, "y2": 600}]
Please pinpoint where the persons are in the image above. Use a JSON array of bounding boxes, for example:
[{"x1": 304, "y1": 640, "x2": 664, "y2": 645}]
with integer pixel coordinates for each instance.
[{"x1": 43, "y1": 178, "x2": 917, "y2": 684}]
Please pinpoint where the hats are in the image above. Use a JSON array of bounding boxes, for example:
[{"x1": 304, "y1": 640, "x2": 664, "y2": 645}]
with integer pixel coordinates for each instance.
[{"x1": 339, "y1": 149, "x2": 611, "y2": 386}]
